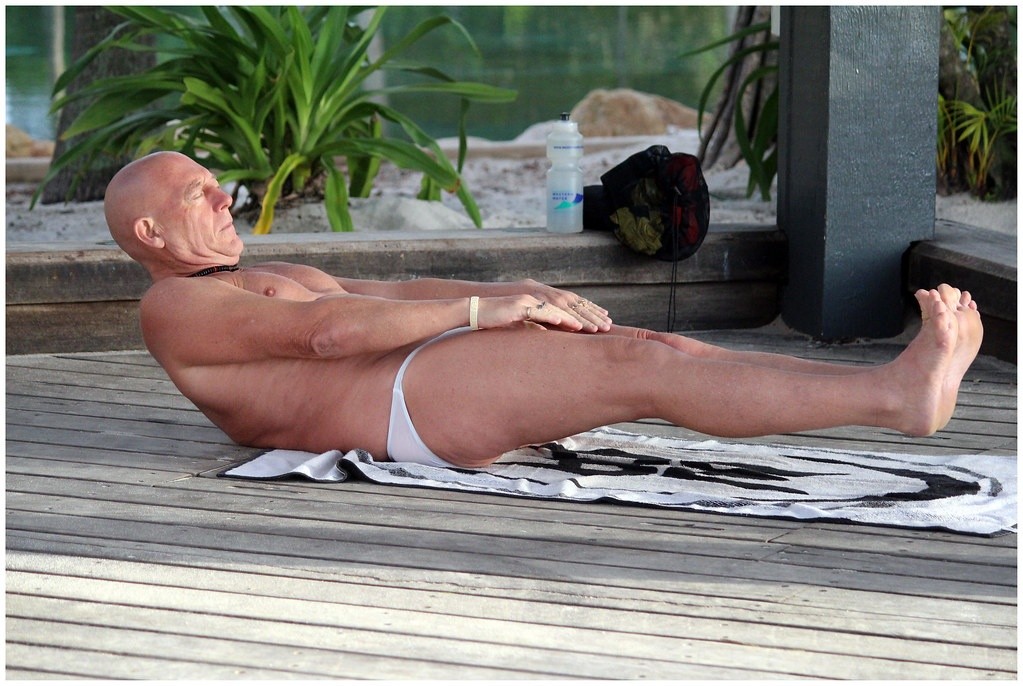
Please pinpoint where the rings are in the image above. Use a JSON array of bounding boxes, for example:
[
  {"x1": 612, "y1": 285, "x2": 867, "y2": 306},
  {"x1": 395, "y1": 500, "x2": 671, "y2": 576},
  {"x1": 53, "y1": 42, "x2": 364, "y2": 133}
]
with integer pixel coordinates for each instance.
[
  {"x1": 584, "y1": 298, "x2": 589, "y2": 304},
  {"x1": 578, "y1": 300, "x2": 584, "y2": 305},
  {"x1": 526, "y1": 306, "x2": 531, "y2": 320},
  {"x1": 536, "y1": 304, "x2": 543, "y2": 310},
  {"x1": 541, "y1": 301, "x2": 547, "y2": 305},
  {"x1": 571, "y1": 305, "x2": 578, "y2": 310}
]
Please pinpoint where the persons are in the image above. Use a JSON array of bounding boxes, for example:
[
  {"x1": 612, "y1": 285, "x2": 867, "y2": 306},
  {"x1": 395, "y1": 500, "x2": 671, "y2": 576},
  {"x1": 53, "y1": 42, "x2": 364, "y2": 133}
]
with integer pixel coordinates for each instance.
[{"x1": 105, "y1": 151, "x2": 984, "y2": 470}]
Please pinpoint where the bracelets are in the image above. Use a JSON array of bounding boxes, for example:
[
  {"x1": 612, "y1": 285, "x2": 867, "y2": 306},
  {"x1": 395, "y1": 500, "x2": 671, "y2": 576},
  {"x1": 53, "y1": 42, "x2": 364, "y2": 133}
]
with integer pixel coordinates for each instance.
[{"x1": 470, "y1": 296, "x2": 479, "y2": 330}]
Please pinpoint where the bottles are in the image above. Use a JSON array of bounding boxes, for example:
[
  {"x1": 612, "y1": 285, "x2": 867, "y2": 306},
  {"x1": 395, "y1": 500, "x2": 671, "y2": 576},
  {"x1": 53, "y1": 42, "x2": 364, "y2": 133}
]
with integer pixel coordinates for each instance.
[{"x1": 544, "y1": 112, "x2": 583, "y2": 234}]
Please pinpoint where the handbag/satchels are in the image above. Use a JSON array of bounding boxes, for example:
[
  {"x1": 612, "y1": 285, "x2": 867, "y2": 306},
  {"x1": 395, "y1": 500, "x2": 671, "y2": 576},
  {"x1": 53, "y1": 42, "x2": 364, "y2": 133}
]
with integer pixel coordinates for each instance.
[{"x1": 583, "y1": 145, "x2": 711, "y2": 262}]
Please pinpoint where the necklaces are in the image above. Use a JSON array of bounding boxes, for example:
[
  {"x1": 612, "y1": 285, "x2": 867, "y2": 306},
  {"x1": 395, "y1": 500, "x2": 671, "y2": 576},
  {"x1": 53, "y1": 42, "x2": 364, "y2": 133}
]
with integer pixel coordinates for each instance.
[{"x1": 186, "y1": 263, "x2": 239, "y2": 278}]
